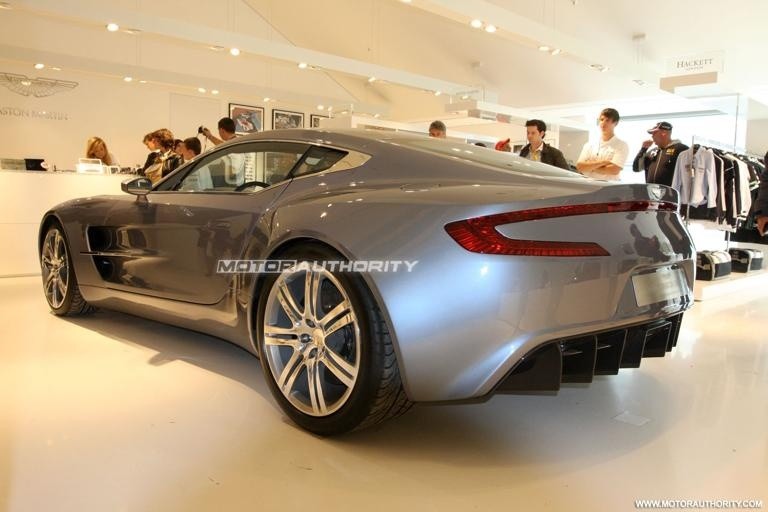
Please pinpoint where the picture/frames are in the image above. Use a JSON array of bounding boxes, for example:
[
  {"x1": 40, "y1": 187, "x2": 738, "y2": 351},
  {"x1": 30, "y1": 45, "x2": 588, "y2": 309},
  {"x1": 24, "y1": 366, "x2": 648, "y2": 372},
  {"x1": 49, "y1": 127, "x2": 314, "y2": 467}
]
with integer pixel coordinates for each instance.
[
  {"x1": 270, "y1": 107, "x2": 305, "y2": 133},
  {"x1": 226, "y1": 102, "x2": 265, "y2": 136},
  {"x1": 308, "y1": 113, "x2": 333, "y2": 128},
  {"x1": 262, "y1": 151, "x2": 298, "y2": 184}
]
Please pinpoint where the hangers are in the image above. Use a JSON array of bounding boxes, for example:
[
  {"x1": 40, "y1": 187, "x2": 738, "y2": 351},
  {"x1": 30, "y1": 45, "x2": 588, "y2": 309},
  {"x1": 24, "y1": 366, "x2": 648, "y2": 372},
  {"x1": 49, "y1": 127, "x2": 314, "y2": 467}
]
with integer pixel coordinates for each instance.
[{"x1": 690, "y1": 136, "x2": 761, "y2": 163}]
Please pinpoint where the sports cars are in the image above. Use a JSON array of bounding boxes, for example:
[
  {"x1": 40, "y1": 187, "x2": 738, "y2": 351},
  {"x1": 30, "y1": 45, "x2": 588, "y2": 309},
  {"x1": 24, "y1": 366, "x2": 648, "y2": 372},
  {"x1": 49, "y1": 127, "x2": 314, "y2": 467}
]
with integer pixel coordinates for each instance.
[{"x1": 37, "y1": 125, "x2": 697, "y2": 438}]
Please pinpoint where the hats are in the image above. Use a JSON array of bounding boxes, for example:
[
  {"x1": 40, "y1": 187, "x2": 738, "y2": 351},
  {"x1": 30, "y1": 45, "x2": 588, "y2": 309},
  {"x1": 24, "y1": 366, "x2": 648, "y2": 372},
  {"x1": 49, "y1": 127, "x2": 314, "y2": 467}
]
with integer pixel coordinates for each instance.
[
  {"x1": 495, "y1": 138, "x2": 511, "y2": 151},
  {"x1": 647, "y1": 122, "x2": 672, "y2": 134}
]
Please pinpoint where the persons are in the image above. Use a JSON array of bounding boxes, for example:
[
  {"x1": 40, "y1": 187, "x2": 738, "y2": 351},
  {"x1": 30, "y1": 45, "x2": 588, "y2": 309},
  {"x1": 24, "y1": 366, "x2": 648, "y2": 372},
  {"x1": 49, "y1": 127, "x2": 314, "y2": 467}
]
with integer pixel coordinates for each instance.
[
  {"x1": 576, "y1": 108, "x2": 629, "y2": 181},
  {"x1": 428, "y1": 120, "x2": 446, "y2": 139},
  {"x1": 520, "y1": 119, "x2": 570, "y2": 170},
  {"x1": 137, "y1": 129, "x2": 214, "y2": 192},
  {"x1": 656, "y1": 210, "x2": 692, "y2": 260},
  {"x1": 631, "y1": 224, "x2": 672, "y2": 263},
  {"x1": 754, "y1": 152, "x2": 768, "y2": 237},
  {"x1": 203, "y1": 118, "x2": 245, "y2": 188},
  {"x1": 87, "y1": 137, "x2": 119, "y2": 166},
  {"x1": 632, "y1": 122, "x2": 690, "y2": 186},
  {"x1": 496, "y1": 138, "x2": 511, "y2": 152}
]
